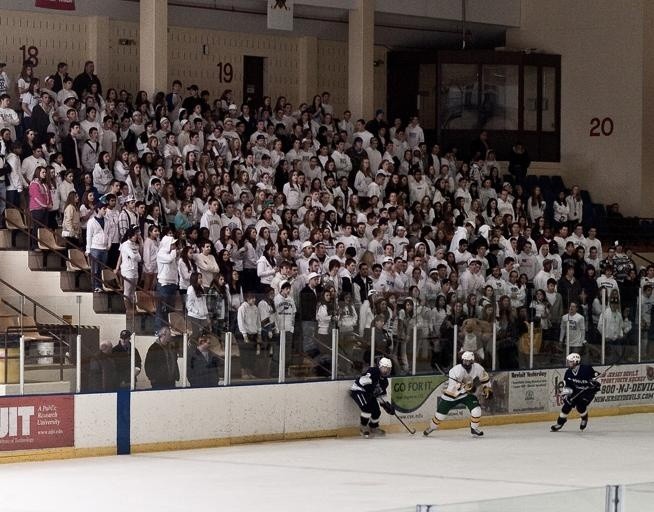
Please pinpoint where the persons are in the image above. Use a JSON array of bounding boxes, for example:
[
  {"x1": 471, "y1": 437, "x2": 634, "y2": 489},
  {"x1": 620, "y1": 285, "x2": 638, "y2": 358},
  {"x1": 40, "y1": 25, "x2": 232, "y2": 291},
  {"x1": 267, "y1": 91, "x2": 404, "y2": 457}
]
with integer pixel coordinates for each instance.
[
  {"x1": 351, "y1": 358, "x2": 395, "y2": 435},
  {"x1": 423, "y1": 352, "x2": 492, "y2": 436},
  {"x1": 551, "y1": 353, "x2": 606, "y2": 431},
  {"x1": 1, "y1": 62, "x2": 652, "y2": 392}
]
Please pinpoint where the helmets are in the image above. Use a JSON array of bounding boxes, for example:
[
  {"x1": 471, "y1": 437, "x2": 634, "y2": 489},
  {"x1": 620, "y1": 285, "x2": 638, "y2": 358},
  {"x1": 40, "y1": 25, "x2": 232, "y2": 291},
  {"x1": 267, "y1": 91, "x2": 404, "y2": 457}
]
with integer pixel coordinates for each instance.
[
  {"x1": 461, "y1": 351, "x2": 475, "y2": 362},
  {"x1": 566, "y1": 353, "x2": 581, "y2": 364},
  {"x1": 378, "y1": 357, "x2": 393, "y2": 370}
]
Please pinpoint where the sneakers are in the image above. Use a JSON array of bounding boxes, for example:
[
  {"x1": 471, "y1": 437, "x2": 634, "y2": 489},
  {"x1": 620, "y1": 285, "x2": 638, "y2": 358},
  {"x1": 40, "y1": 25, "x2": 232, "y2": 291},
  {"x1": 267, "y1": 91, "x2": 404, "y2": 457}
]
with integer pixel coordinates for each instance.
[
  {"x1": 370, "y1": 426, "x2": 385, "y2": 434},
  {"x1": 580, "y1": 420, "x2": 587, "y2": 429},
  {"x1": 360, "y1": 424, "x2": 369, "y2": 435},
  {"x1": 423, "y1": 426, "x2": 433, "y2": 435},
  {"x1": 551, "y1": 424, "x2": 562, "y2": 431},
  {"x1": 471, "y1": 427, "x2": 483, "y2": 435}
]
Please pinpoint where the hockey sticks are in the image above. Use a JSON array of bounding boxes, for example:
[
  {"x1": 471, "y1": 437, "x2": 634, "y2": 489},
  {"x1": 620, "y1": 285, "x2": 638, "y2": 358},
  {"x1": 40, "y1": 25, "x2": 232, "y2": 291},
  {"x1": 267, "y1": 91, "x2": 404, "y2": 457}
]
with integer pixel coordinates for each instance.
[
  {"x1": 570, "y1": 344, "x2": 625, "y2": 401},
  {"x1": 435, "y1": 364, "x2": 464, "y2": 385},
  {"x1": 379, "y1": 396, "x2": 416, "y2": 434}
]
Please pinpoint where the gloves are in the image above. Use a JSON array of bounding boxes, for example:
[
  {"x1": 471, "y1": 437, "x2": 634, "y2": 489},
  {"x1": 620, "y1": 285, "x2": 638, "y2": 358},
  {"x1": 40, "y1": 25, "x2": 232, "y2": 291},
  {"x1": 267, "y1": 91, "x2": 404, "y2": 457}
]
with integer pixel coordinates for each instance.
[
  {"x1": 380, "y1": 400, "x2": 396, "y2": 414},
  {"x1": 482, "y1": 386, "x2": 494, "y2": 400},
  {"x1": 463, "y1": 383, "x2": 476, "y2": 394}
]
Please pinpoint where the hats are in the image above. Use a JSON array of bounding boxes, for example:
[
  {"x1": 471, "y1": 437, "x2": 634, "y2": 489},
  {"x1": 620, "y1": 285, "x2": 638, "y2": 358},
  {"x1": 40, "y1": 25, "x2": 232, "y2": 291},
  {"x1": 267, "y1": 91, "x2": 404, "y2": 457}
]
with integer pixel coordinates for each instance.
[
  {"x1": 278, "y1": 240, "x2": 479, "y2": 297},
  {"x1": 120, "y1": 330, "x2": 131, "y2": 339},
  {"x1": 45, "y1": 76, "x2": 238, "y2": 130}
]
